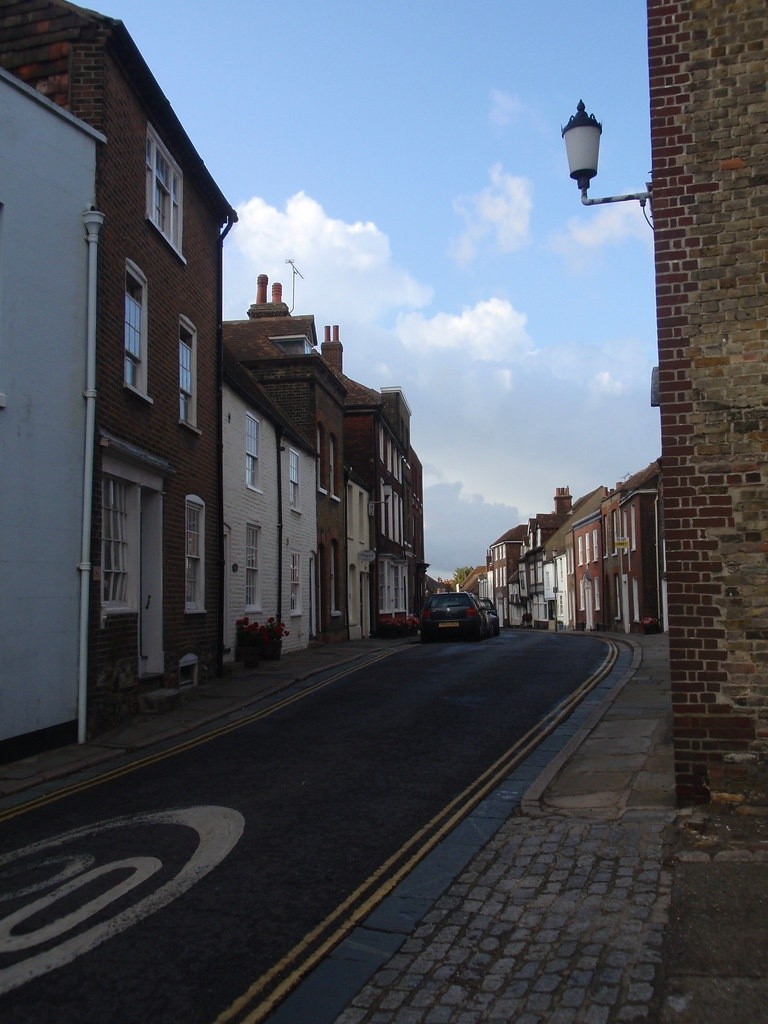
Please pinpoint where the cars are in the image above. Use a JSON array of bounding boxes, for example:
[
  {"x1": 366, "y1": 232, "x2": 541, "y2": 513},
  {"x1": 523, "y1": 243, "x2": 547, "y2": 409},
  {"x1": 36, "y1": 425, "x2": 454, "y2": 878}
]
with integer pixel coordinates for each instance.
[
  {"x1": 421, "y1": 592, "x2": 481, "y2": 643},
  {"x1": 469, "y1": 593, "x2": 501, "y2": 636}
]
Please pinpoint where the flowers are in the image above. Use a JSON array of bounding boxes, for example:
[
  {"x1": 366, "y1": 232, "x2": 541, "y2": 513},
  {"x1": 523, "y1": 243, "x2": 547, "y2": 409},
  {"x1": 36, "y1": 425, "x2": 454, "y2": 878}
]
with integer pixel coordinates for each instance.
[{"x1": 236, "y1": 617, "x2": 289, "y2": 646}]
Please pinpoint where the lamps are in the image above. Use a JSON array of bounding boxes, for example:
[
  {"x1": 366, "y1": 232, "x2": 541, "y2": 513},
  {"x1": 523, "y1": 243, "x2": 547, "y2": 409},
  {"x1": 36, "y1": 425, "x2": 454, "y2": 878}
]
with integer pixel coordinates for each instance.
[{"x1": 561, "y1": 100, "x2": 653, "y2": 230}]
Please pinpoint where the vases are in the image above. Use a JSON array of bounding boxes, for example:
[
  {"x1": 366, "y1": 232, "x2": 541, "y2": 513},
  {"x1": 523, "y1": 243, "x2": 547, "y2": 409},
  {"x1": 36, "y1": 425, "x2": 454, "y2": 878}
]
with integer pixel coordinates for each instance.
[{"x1": 236, "y1": 641, "x2": 282, "y2": 662}]
